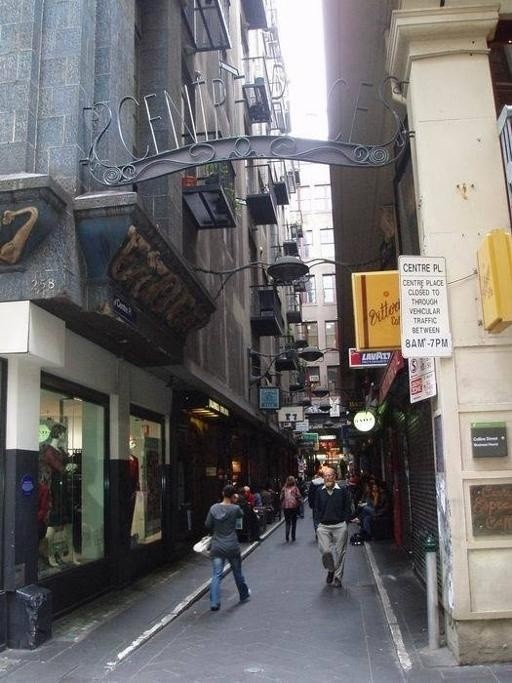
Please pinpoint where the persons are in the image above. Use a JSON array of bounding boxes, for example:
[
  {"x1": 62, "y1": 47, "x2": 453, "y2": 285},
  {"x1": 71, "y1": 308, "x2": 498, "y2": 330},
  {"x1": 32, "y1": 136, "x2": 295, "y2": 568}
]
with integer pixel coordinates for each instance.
[
  {"x1": 126, "y1": 435, "x2": 140, "y2": 533},
  {"x1": 205, "y1": 485, "x2": 251, "y2": 611},
  {"x1": 45, "y1": 424, "x2": 78, "y2": 567},
  {"x1": 313, "y1": 468, "x2": 351, "y2": 588},
  {"x1": 235, "y1": 466, "x2": 387, "y2": 542}
]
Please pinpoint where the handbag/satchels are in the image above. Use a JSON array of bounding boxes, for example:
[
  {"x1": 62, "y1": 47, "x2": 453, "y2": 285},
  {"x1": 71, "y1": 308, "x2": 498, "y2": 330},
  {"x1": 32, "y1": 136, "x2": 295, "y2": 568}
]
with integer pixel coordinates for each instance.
[{"x1": 193, "y1": 534, "x2": 214, "y2": 557}]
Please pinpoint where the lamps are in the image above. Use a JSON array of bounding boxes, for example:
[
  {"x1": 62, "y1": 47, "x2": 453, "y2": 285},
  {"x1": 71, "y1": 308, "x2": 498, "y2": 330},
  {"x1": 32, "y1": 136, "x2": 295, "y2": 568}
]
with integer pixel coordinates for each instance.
[{"x1": 187, "y1": 255, "x2": 331, "y2": 412}]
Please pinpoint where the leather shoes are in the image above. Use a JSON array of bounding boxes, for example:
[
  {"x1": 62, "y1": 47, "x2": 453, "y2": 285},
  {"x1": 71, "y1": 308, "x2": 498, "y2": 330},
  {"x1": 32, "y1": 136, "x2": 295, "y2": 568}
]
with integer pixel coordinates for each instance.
[{"x1": 326, "y1": 572, "x2": 342, "y2": 587}]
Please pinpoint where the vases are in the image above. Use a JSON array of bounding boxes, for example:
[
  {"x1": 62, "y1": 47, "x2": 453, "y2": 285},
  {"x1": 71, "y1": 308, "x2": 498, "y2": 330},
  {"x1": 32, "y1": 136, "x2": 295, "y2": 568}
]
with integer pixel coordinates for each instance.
[{"x1": 183, "y1": 175, "x2": 196, "y2": 186}]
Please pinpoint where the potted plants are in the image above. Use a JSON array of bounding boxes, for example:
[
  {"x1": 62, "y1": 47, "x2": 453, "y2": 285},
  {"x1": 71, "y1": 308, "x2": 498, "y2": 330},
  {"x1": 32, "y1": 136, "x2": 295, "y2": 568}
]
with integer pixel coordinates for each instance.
[{"x1": 204, "y1": 163, "x2": 239, "y2": 222}]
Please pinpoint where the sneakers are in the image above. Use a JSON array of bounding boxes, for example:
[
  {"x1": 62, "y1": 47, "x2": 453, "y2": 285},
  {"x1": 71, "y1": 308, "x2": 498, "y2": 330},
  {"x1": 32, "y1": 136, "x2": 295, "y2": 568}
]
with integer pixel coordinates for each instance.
[
  {"x1": 211, "y1": 602, "x2": 220, "y2": 611},
  {"x1": 240, "y1": 589, "x2": 251, "y2": 601}
]
